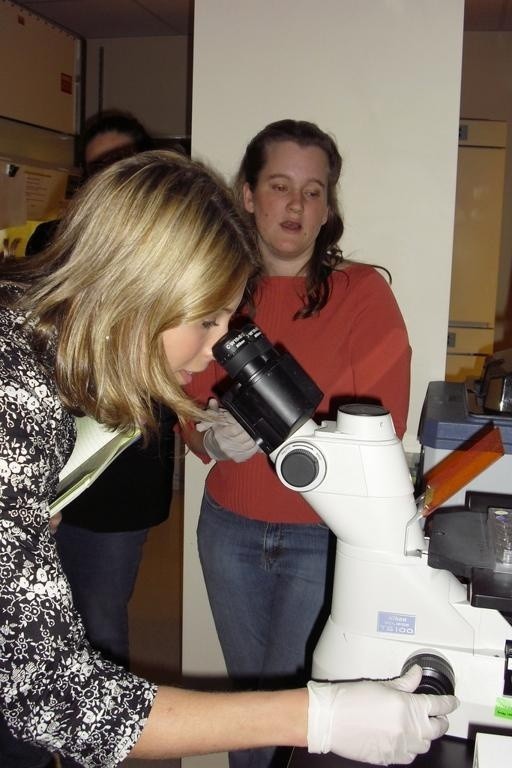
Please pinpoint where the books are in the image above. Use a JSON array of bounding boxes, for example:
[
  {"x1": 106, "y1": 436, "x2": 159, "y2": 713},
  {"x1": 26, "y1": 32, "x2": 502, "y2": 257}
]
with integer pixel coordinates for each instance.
[{"x1": 41, "y1": 407, "x2": 152, "y2": 524}]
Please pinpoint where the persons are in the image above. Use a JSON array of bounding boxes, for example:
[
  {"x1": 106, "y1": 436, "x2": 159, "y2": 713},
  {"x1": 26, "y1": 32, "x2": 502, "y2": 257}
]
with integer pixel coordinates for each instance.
[
  {"x1": 0, "y1": 148, "x2": 461, "y2": 767},
  {"x1": 168, "y1": 117, "x2": 416, "y2": 768},
  {"x1": 22, "y1": 106, "x2": 178, "y2": 677}
]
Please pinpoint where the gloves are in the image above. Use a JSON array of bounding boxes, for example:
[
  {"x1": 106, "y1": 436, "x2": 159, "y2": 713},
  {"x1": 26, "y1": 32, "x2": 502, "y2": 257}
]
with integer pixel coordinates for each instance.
[
  {"x1": 196, "y1": 399, "x2": 265, "y2": 465},
  {"x1": 306, "y1": 665, "x2": 460, "y2": 767}
]
